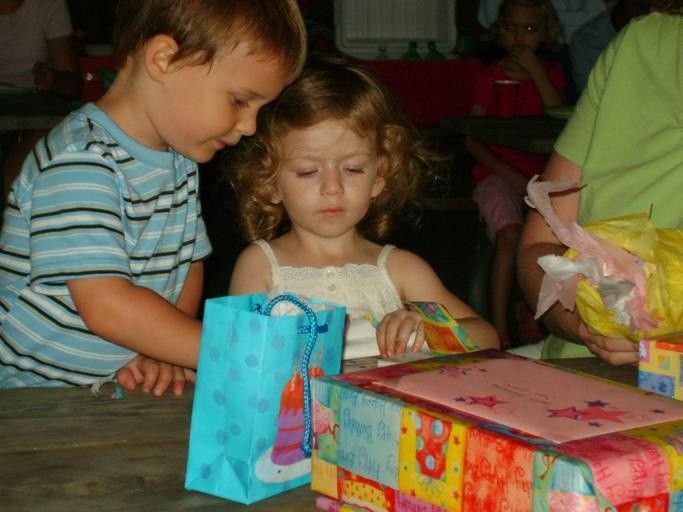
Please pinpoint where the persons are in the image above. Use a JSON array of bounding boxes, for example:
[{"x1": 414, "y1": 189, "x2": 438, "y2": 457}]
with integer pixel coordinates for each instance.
[
  {"x1": 1, "y1": 1, "x2": 75, "y2": 93},
  {"x1": 219, "y1": 52, "x2": 503, "y2": 361},
  {"x1": 510, "y1": 2, "x2": 682, "y2": 370},
  {"x1": 475, "y1": 2, "x2": 617, "y2": 98},
  {"x1": 1, "y1": 1, "x2": 308, "y2": 399},
  {"x1": 460, "y1": 1, "x2": 573, "y2": 349}
]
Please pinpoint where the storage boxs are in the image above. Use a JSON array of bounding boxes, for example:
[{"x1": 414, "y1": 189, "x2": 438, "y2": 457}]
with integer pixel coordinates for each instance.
[
  {"x1": 635, "y1": 330, "x2": 682, "y2": 403},
  {"x1": 308, "y1": 354, "x2": 682, "y2": 511}
]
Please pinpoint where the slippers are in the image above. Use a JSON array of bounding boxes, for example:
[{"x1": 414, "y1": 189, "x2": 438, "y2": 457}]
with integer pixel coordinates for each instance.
[
  {"x1": 497, "y1": 335, "x2": 512, "y2": 351},
  {"x1": 514, "y1": 301, "x2": 547, "y2": 345}
]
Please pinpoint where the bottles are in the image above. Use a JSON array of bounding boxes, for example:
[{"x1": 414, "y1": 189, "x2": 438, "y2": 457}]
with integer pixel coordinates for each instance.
[
  {"x1": 370, "y1": 38, "x2": 446, "y2": 62},
  {"x1": 78, "y1": 41, "x2": 121, "y2": 107}
]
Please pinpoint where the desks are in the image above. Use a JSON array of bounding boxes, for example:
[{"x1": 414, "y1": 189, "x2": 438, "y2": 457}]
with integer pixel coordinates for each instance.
[{"x1": 0, "y1": 351, "x2": 683, "y2": 510}]
